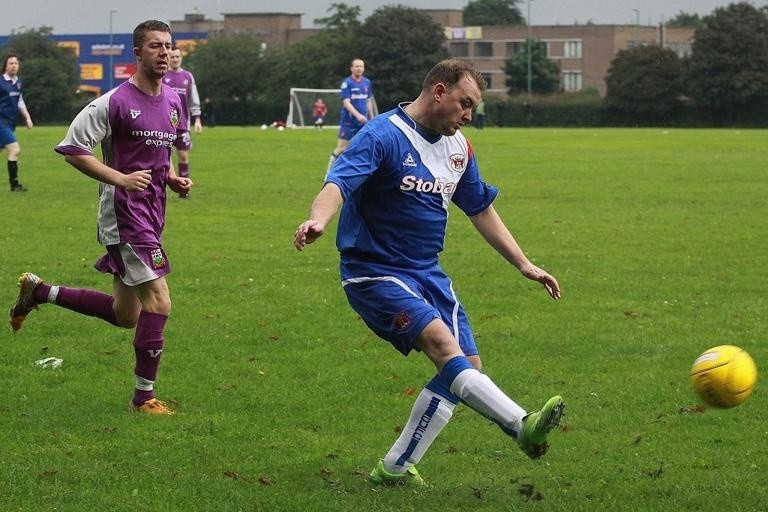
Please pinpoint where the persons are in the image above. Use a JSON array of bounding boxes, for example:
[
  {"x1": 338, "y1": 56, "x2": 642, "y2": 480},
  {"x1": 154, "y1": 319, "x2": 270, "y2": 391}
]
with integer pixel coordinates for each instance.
[
  {"x1": 324, "y1": 58, "x2": 374, "y2": 186},
  {"x1": 165, "y1": 47, "x2": 202, "y2": 198},
  {"x1": 0, "y1": 55, "x2": 33, "y2": 191},
  {"x1": 297, "y1": 57, "x2": 564, "y2": 487},
  {"x1": 521, "y1": 99, "x2": 536, "y2": 125},
  {"x1": 10, "y1": 20, "x2": 194, "y2": 417},
  {"x1": 497, "y1": 96, "x2": 508, "y2": 127},
  {"x1": 475, "y1": 97, "x2": 484, "y2": 128},
  {"x1": 313, "y1": 98, "x2": 327, "y2": 129}
]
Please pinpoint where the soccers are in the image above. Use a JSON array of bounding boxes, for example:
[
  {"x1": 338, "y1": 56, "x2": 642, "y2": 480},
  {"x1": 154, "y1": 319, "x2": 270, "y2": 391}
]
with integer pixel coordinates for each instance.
[{"x1": 691, "y1": 343, "x2": 757, "y2": 409}]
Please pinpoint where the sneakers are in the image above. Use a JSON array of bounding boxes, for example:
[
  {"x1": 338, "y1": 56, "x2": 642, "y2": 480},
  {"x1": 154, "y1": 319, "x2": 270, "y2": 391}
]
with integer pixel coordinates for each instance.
[
  {"x1": 9, "y1": 272, "x2": 42, "y2": 330},
  {"x1": 367, "y1": 459, "x2": 432, "y2": 493},
  {"x1": 128, "y1": 398, "x2": 176, "y2": 415},
  {"x1": 518, "y1": 396, "x2": 565, "y2": 460}
]
